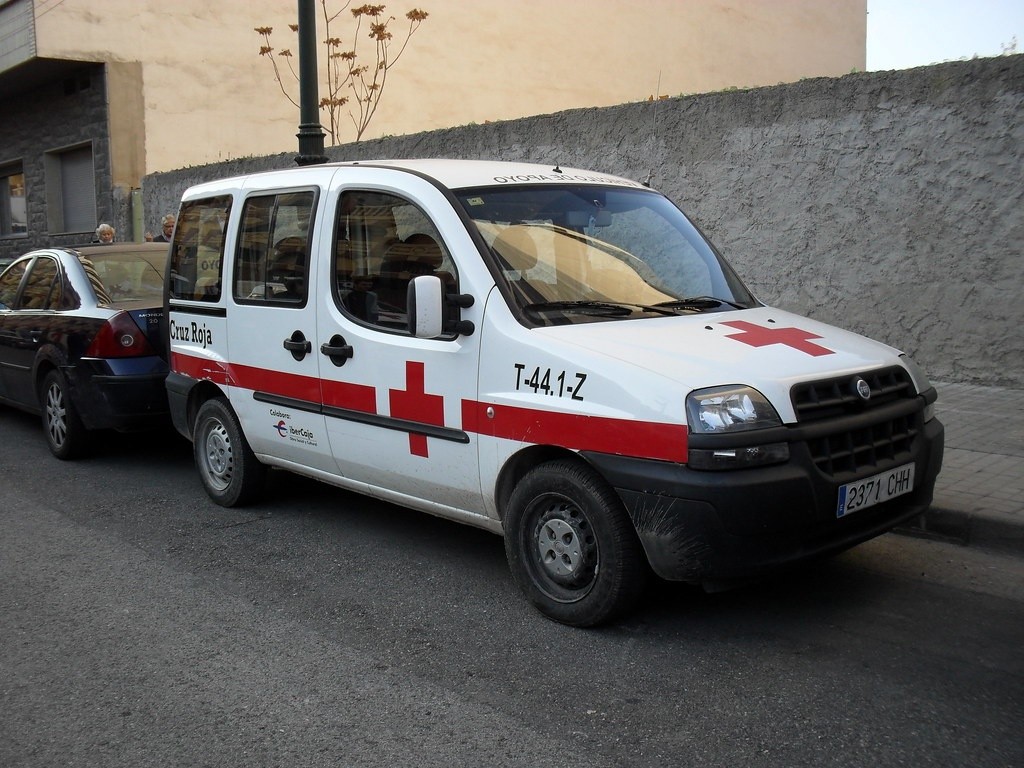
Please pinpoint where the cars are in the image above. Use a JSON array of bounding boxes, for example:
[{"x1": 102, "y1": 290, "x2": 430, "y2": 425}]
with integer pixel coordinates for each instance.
[{"x1": 0, "y1": 242, "x2": 222, "y2": 460}]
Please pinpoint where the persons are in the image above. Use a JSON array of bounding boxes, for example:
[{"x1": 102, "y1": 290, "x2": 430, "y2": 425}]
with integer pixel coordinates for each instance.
[
  {"x1": 144, "y1": 214, "x2": 175, "y2": 242},
  {"x1": 88, "y1": 224, "x2": 116, "y2": 243}
]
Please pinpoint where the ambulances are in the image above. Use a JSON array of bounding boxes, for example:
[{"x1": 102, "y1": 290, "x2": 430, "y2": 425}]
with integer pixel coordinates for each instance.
[{"x1": 162, "y1": 155, "x2": 946, "y2": 630}]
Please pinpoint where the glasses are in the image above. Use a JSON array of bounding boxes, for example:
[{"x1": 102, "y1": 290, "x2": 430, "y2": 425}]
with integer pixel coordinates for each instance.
[{"x1": 163, "y1": 224, "x2": 174, "y2": 228}]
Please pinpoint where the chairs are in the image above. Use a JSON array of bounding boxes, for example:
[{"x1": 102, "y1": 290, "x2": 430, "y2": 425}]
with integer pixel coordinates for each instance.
[
  {"x1": 494, "y1": 227, "x2": 563, "y2": 318},
  {"x1": 396, "y1": 233, "x2": 457, "y2": 319},
  {"x1": 140, "y1": 262, "x2": 165, "y2": 298},
  {"x1": 104, "y1": 265, "x2": 136, "y2": 300},
  {"x1": 270, "y1": 235, "x2": 307, "y2": 298}
]
